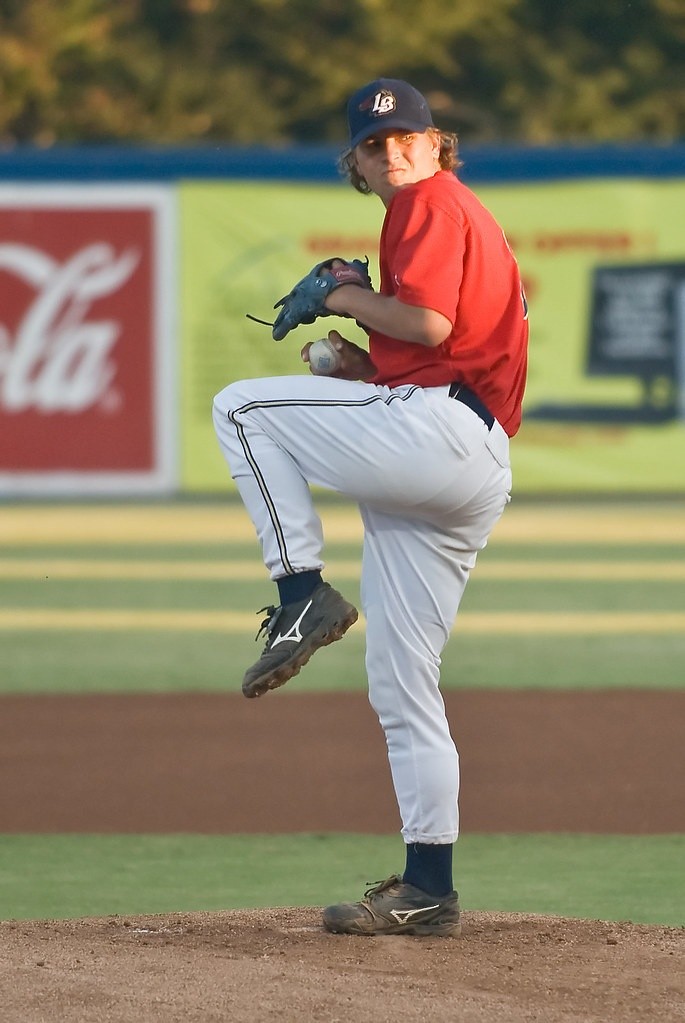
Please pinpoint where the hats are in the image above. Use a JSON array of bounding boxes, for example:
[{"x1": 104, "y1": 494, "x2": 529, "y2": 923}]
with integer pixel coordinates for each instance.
[{"x1": 347, "y1": 78, "x2": 435, "y2": 149}]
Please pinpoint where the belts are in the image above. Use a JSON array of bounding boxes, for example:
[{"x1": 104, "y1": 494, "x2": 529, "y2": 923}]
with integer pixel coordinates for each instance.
[{"x1": 449, "y1": 382, "x2": 495, "y2": 430}]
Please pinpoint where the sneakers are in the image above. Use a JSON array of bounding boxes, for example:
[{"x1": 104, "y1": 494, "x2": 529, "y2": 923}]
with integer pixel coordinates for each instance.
[
  {"x1": 323, "y1": 874, "x2": 461, "y2": 938},
  {"x1": 242, "y1": 582, "x2": 358, "y2": 698}
]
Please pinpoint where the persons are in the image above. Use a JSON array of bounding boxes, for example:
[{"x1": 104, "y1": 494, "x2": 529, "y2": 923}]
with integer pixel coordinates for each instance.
[{"x1": 210, "y1": 79, "x2": 528, "y2": 938}]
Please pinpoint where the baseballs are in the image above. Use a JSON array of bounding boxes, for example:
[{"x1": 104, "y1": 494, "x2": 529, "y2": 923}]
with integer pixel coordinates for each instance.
[{"x1": 309, "y1": 338, "x2": 342, "y2": 376}]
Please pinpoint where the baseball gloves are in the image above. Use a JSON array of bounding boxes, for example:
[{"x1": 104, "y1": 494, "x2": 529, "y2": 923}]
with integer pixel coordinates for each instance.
[{"x1": 271, "y1": 255, "x2": 374, "y2": 341}]
